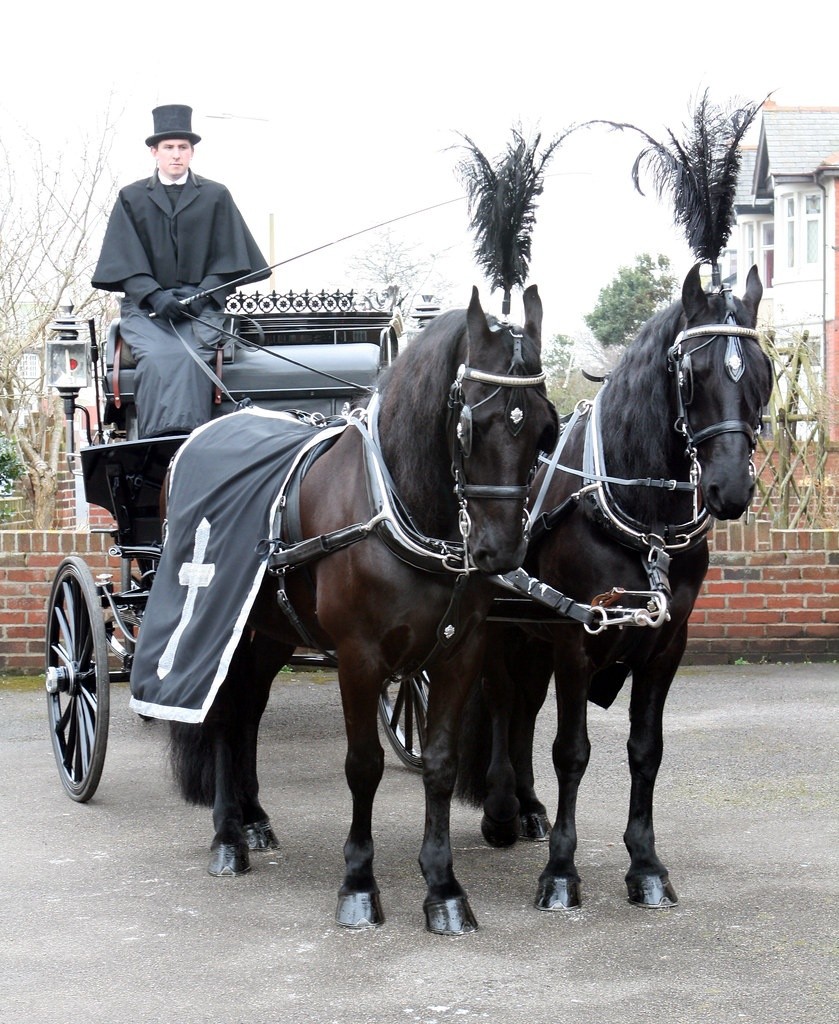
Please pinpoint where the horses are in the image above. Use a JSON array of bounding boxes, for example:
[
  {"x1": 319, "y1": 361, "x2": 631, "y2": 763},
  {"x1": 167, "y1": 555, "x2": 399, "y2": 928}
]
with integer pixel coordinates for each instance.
[
  {"x1": 158, "y1": 285, "x2": 555, "y2": 937},
  {"x1": 420, "y1": 259, "x2": 773, "y2": 916}
]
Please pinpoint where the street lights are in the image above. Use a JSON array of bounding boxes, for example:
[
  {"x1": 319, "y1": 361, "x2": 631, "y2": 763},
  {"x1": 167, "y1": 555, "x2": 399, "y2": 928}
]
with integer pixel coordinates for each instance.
[{"x1": 44, "y1": 304, "x2": 98, "y2": 527}]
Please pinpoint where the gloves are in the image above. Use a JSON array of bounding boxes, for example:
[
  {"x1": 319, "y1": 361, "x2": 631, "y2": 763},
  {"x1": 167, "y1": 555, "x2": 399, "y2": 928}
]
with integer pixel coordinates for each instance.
[
  {"x1": 175, "y1": 287, "x2": 209, "y2": 318},
  {"x1": 147, "y1": 288, "x2": 189, "y2": 324}
]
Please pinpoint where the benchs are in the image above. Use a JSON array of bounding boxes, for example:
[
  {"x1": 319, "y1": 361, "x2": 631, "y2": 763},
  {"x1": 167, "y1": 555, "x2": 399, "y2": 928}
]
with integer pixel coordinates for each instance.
[{"x1": 106, "y1": 321, "x2": 400, "y2": 425}]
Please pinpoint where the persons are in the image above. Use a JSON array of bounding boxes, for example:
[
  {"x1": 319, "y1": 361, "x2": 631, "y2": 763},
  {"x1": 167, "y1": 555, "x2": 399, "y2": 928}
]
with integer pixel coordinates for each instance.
[{"x1": 91, "y1": 105, "x2": 272, "y2": 440}]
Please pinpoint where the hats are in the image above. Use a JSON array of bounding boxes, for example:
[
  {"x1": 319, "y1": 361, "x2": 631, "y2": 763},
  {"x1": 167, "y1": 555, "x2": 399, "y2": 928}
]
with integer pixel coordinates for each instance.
[{"x1": 146, "y1": 106, "x2": 200, "y2": 148}]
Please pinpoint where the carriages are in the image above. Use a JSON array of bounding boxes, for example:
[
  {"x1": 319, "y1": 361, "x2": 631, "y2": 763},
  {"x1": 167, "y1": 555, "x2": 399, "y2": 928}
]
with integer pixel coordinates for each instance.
[{"x1": 42, "y1": 262, "x2": 777, "y2": 928}]
